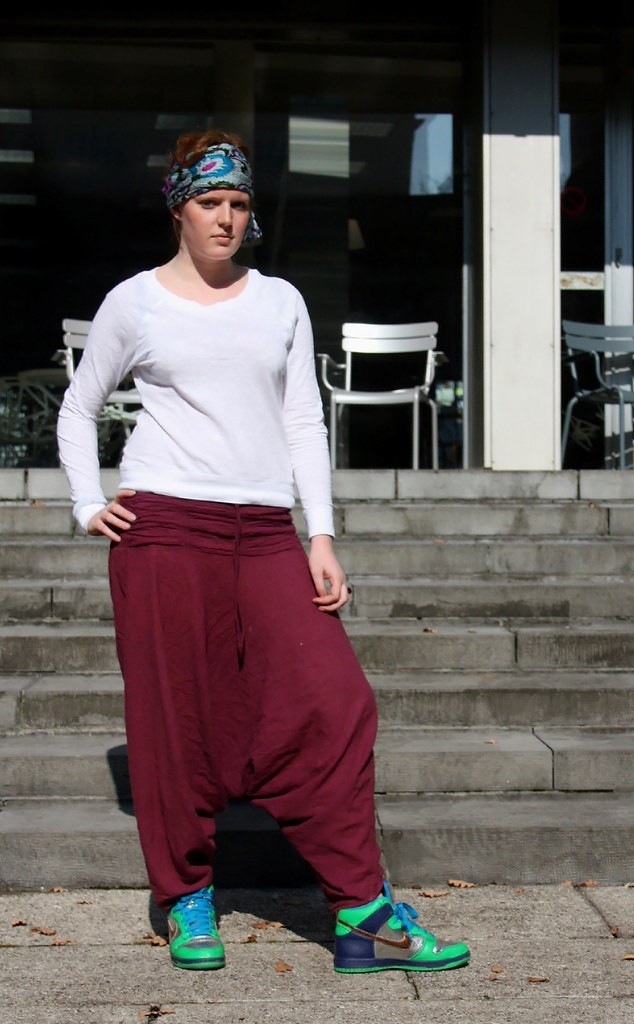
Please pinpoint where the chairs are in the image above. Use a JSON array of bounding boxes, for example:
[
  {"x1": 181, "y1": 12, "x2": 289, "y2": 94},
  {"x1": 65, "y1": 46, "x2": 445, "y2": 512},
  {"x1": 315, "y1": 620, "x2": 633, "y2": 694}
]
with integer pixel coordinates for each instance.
[
  {"x1": 316, "y1": 321, "x2": 440, "y2": 470},
  {"x1": 561, "y1": 321, "x2": 634, "y2": 471},
  {"x1": 62, "y1": 318, "x2": 142, "y2": 437},
  {"x1": 0, "y1": 377, "x2": 61, "y2": 466}
]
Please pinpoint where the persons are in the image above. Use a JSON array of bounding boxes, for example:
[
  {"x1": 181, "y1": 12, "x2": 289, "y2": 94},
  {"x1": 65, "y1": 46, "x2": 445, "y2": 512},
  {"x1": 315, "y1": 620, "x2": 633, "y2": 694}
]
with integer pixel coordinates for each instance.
[
  {"x1": 348, "y1": 172, "x2": 451, "y2": 468},
  {"x1": 56, "y1": 128, "x2": 470, "y2": 973}
]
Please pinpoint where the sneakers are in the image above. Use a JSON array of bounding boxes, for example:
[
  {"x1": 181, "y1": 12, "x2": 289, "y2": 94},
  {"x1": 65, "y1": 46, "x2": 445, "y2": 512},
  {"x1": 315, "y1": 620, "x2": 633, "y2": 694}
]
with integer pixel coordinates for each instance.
[
  {"x1": 333, "y1": 879, "x2": 471, "y2": 973},
  {"x1": 165, "y1": 882, "x2": 226, "y2": 971}
]
